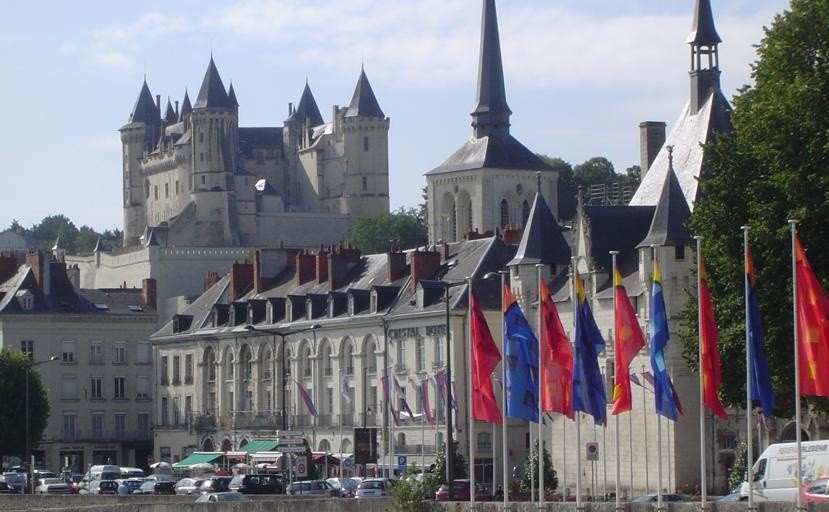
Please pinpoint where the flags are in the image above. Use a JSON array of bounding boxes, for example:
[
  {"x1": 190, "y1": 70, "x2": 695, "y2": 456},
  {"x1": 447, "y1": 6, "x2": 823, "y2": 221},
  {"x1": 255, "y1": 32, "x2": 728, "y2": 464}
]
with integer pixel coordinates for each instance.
[
  {"x1": 786, "y1": 218, "x2": 829, "y2": 396},
  {"x1": 693, "y1": 235, "x2": 733, "y2": 423},
  {"x1": 380, "y1": 366, "x2": 461, "y2": 428},
  {"x1": 740, "y1": 225, "x2": 777, "y2": 419},
  {"x1": 533, "y1": 261, "x2": 579, "y2": 422},
  {"x1": 341, "y1": 368, "x2": 355, "y2": 405},
  {"x1": 646, "y1": 239, "x2": 686, "y2": 425},
  {"x1": 608, "y1": 248, "x2": 646, "y2": 416},
  {"x1": 570, "y1": 251, "x2": 609, "y2": 428},
  {"x1": 288, "y1": 375, "x2": 320, "y2": 419},
  {"x1": 467, "y1": 274, "x2": 504, "y2": 427},
  {"x1": 499, "y1": 263, "x2": 563, "y2": 428}
]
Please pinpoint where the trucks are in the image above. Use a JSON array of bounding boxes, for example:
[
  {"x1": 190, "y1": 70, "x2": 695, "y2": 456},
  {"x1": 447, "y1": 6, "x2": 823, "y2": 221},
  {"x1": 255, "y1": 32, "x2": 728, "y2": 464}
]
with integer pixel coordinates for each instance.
[{"x1": 739, "y1": 439, "x2": 829, "y2": 503}]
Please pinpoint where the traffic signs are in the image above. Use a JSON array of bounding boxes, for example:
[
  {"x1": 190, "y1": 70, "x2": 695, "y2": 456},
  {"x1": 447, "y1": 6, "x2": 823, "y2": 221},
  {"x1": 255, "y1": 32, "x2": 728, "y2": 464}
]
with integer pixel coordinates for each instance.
[
  {"x1": 276, "y1": 446, "x2": 306, "y2": 453},
  {"x1": 275, "y1": 429, "x2": 304, "y2": 436},
  {"x1": 275, "y1": 438, "x2": 304, "y2": 445}
]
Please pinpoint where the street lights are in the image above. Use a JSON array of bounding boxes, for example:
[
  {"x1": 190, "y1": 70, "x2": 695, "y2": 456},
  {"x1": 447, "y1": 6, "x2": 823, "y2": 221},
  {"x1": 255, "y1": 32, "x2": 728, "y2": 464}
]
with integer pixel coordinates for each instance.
[
  {"x1": 22, "y1": 354, "x2": 60, "y2": 491},
  {"x1": 441, "y1": 268, "x2": 504, "y2": 486},
  {"x1": 243, "y1": 321, "x2": 325, "y2": 496}
]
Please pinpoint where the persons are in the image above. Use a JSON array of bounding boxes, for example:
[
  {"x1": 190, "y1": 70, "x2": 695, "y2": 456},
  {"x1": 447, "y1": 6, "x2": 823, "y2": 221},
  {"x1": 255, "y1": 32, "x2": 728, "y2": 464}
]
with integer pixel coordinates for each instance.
[{"x1": 116, "y1": 481, "x2": 130, "y2": 495}]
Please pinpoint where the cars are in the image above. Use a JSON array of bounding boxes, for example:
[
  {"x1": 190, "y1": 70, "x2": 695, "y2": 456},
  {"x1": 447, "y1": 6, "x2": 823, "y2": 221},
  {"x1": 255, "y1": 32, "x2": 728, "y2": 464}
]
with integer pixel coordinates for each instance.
[
  {"x1": 430, "y1": 476, "x2": 493, "y2": 503},
  {"x1": 715, "y1": 482, "x2": 741, "y2": 502},
  {"x1": 793, "y1": 475, "x2": 829, "y2": 512},
  {"x1": 187, "y1": 490, "x2": 252, "y2": 502},
  {"x1": 629, "y1": 491, "x2": 691, "y2": 502}
]
